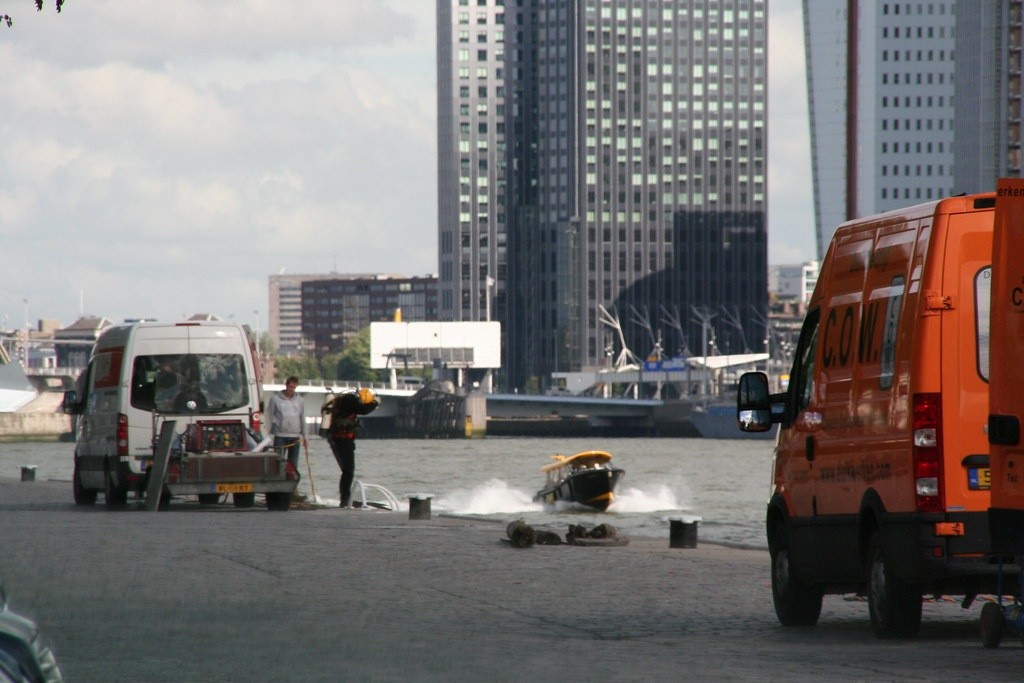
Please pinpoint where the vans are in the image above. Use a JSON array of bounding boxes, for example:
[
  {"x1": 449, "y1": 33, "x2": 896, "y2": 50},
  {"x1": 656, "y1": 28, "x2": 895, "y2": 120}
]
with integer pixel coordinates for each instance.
[
  {"x1": 63, "y1": 318, "x2": 266, "y2": 508},
  {"x1": 738, "y1": 173, "x2": 1024, "y2": 650}
]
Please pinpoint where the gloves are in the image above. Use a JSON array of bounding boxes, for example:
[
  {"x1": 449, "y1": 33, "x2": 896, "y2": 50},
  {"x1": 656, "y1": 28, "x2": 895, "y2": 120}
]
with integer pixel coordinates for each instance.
[{"x1": 374, "y1": 395, "x2": 381, "y2": 403}]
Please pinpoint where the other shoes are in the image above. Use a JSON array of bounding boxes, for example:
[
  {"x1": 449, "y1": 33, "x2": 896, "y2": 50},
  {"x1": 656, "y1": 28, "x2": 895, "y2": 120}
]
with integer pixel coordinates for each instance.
[
  {"x1": 340, "y1": 499, "x2": 363, "y2": 508},
  {"x1": 292, "y1": 494, "x2": 307, "y2": 502}
]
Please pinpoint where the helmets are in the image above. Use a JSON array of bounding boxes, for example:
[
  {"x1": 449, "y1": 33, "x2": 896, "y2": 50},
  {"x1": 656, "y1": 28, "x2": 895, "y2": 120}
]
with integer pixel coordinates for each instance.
[{"x1": 356, "y1": 390, "x2": 373, "y2": 405}]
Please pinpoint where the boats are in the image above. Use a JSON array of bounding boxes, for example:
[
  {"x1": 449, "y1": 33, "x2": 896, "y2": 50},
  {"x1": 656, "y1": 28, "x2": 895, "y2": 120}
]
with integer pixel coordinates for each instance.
[
  {"x1": 532, "y1": 448, "x2": 627, "y2": 510},
  {"x1": 393, "y1": 357, "x2": 490, "y2": 439}
]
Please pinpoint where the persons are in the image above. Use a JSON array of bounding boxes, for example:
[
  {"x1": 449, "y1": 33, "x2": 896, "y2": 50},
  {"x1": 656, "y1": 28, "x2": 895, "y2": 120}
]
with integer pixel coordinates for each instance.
[
  {"x1": 266, "y1": 376, "x2": 309, "y2": 511},
  {"x1": 319, "y1": 386, "x2": 381, "y2": 510}
]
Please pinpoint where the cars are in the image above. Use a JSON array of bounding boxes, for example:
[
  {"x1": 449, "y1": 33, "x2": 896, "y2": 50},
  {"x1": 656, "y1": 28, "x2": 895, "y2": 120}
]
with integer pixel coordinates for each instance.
[{"x1": 547, "y1": 386, "x2": 572, "y2": 396}]
